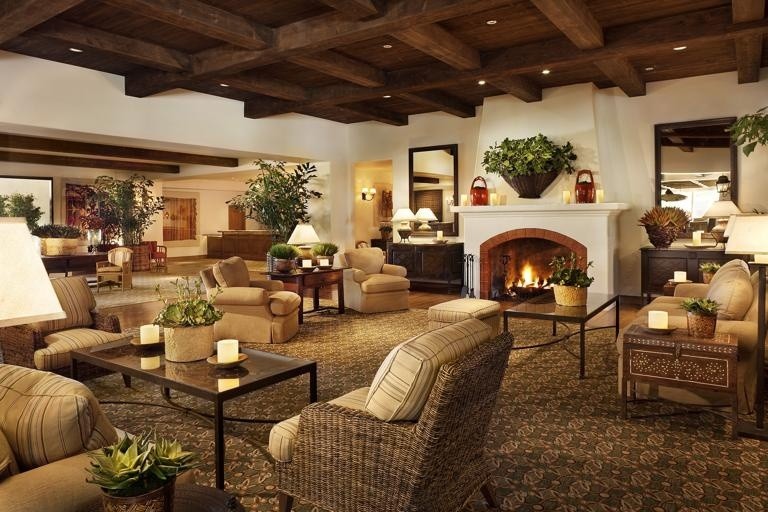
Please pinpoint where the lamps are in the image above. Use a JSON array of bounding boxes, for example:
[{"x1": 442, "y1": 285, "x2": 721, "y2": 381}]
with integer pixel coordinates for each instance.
[
  {"x1": 704, "y1": 200, "x2": 740, "y2": 237},
  {"x1": 660, "y1": 188, "x2": 687, "y2": 202},
  {"x1": 288, "y1": 225, "x2": 320, "y2": 252},
  {"x1": 414, "y1": 208, "x2": 438, "y2": 231},
  {"x1": 1, "y1": 216, "x2": 68, "y2": 328},
  {"x1": 716, "y1": 172, "x2": 730, "y2": 197},
  {"x1": 362, "y1": 188, "x2": 376, "y2": 201},
  {"x1": 724, "y1": 213, "x2": 768, "y2": 440},
  {"x1": 390, "y1": 208, "x2": 416, "y2": 244}
]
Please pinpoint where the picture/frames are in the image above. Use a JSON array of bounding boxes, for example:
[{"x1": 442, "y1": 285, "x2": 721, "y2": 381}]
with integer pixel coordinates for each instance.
[{"x1": 373, "y1": 183, "x2": 392, "y2": 225}]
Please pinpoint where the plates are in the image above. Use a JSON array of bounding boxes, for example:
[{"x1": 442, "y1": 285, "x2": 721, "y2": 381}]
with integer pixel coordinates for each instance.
[
  {"x1": 207, "y1": 352, "x2": 248, "y2": 368},
  {"x1": 316, "y1": 265, "x2": 333, "y2": 270},
  {"x1": 668, "y1": 279, "x2": 693, "y2": 285},
  {"x1": 433, "y1": 239, "x2": 449, "y2": 244},
  {"x1": 207, "y1": 366, "x2": 250, "y2": 378},
  {"x1": 298, "y1": 266, "x2": 316, "y2": 273},
  {"x1": 130, "y1": 336, "x2": 164, "y2": 349},
  {"x1": 638, "y1": 323, "x2": 679, "y2": 335},
  {"x1": 133, "y1": 347, "x2": 165, "y2": 357},
  {"x1": 683, "y1": 242, "x2": 717, "y2": 249}
]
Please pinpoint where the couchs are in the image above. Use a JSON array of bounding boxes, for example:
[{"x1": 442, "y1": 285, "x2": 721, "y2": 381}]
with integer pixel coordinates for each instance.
[
  {"x1": 197, "y1": 256, "x2": 301, "y2": 343},
  {"x1": 336, "y1": 246, "x2": 410, "y2": 314},
  {"x1": 617, "y1": 259, "x2": 768, "y2": 415},
  {"x1": 1, "y1": 245, "x2": 191, "y2": 512}
]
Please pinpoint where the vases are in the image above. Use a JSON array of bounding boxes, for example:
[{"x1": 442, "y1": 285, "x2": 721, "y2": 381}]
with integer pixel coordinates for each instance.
[{"x1": 644, "y1": 225, "x2": 679, "y2": 249}]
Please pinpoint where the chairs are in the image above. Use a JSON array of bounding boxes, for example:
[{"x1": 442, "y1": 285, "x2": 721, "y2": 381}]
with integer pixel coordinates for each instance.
[{"x1": 267, "y1": 318, "x2": 513, "y2": 512}]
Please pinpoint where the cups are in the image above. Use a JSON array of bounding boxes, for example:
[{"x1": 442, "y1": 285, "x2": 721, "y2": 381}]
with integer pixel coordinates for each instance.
[
  {"x1": 437, "y1": 230, "x2": 443, "y2": 241},
  {"x1": 216, "y1": 337, "x2": 239, "y2": 362},
  {"x1": 302, "y1": 259, "x2": 312, "y2": 267},
  {"x1": 562, "y1": 190, "x2": 571, "y2": 204},
  {"x1": 319, "y1": 259, "x2": 329, "y2": 266},
  {"x1": 489, "y1": 192, "x2": 498, "y2": 206},
  {"x1": 692, "y1": 231, "x2": 702, "y2": 246},
  {"x1": 595, "y1": 189, "x2": 605, "y2": 203},
  {"x1": 218, "y1": 378, "x2": 240, "y2": 392},
  {"x1": 140, "y1": 324, "x2": 160, "y2": 343},
  {"x1": 141, "y1": 357, "x2": 160, "y2": 371}
]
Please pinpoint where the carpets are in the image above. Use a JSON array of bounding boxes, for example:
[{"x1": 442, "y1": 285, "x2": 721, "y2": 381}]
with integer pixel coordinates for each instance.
[{"x1": 89, "y1": 296, "x2": 768, "y2": 512}]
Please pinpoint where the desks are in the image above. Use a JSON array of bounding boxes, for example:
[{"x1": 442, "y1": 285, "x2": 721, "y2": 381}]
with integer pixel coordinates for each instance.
[
  {"x1": 385, "y1": 242, "x2": 464, "y2": 295},
  {"x1": 371, "y1": 239, "x2": 393, "y2": 264}
]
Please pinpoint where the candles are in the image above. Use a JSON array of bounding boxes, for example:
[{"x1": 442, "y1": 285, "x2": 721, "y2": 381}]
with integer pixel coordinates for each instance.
[
  {"x1": 299, "y1": 259, "x2": 316, "y2": 272},
  {"x1": 490, "y1": 193, "x2": 507, "y2": 205},
  {"x1": 692, "y1": 229, "x2": 701, "y2": 246},
  {"x1": 562, "y1": 186, "x2": 570, "y2": 205},
  {"x1": 317, "y1": 258, "x2": 333, "y2": 270},
  {"x1": 437, "y1": 230, "x2": 444, "y2": 241},
  {"x1": 134, "y1": 342, "x2": 165, "y2": 370},
  {"x1": 131, "y1": 323, "x2": 165, "y2": 346},
  {"x1": 205, "y1": 337, "x2": 248, "y2": 368},
  {"x1": 208, "y1": 367, "x2": 250, "y2": 393},
  {"x1": 596, "y1": 185, "x2": 604, "y2": 202}
]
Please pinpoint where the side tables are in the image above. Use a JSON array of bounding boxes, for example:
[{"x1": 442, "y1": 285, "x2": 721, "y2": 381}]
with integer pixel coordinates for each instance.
[
  {"x1": 621, "y1": 325, "x2": 739, "y2": 441},
  {"x1": 663, "y1": 281, "x2": 690, "y2": 296},
  {"x1": 73, "y1": 487, "x2": 246, "y2": 511}
]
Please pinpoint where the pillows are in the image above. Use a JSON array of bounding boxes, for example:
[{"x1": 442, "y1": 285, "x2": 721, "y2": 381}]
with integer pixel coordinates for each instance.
[{"x1": 706, "y1": 257, "x2": 754, "y2": 321}]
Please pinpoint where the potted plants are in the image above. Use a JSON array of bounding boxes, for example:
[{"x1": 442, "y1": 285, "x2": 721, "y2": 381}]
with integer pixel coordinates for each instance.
[
  {"x1": 31, "y1": 173, "x2": 170, "y2": 271},
  {"x1": 547, "y1": 250, "x2": 594, "y2": 307},
  {"x1": 152, "y1": 276, "x2": 228, "y2": 363},
  {"x1": 481, "y1": 131, "x2": 577, "y2": 198},
  {"x1": 315, "y1": 242, "x2": 339, "y2": 265},
  {"x1": 225, "y1": 157, "x2": 323, "y2": 281},
  {"x1": 268, "y1": 245, "x2": 296, "y2": 271},
  {"x1": 698, "y1": 261, "x2": 721, "y2": 284},
  {"x1": 378, "y1": 222, "x2": 392, "y2": 241},
  {"x1": 82, "y1": 430, "x2": 206, "y2": 512},
  {"x1": 680, "y1": 296, "x2": 721, "y2": 338}
]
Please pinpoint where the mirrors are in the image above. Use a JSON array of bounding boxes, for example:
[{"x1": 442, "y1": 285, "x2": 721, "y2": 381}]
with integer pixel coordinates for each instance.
[
  {"x1": 408, "y1": 144, "x2": 459, "y2": 236},
  {"x1": 654, "y1": 117, "x2": 737, "y2": 239}
]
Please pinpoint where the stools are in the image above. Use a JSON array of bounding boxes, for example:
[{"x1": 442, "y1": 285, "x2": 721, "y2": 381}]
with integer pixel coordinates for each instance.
[{"x1": 427, "y1": 297, "x2": 500, "y2": 340}]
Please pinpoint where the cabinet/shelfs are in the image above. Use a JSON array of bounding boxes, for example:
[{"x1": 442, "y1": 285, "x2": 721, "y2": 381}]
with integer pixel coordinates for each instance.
[{"x1": 639, "y1": 244, "x2": 754, "y2": 309}]
